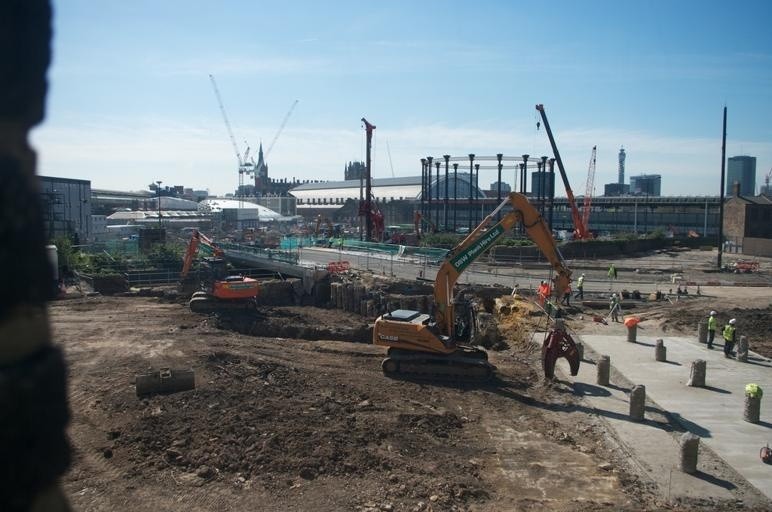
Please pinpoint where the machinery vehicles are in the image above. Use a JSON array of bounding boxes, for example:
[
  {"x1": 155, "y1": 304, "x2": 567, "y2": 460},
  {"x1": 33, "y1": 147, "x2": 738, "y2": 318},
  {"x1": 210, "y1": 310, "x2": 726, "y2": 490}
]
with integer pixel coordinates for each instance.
[
  {"x1": 313, "y1": 212, "x2": 340, "y2": 241},
  {"x1": 371, "y1": 192, "x2": 587, "y2": 386},
  {"x1": 394, "y1": 209, "x2": 437, "y2": 249},
  {"x1": 176, "y1": 225, "x2": 260, "y2": 315},
  {"x1": 532, "y1": 101, "x2": 599, "y2": 241},
  {"x1": 728, "y1": 258, "x2": 760, "y2": 273}
]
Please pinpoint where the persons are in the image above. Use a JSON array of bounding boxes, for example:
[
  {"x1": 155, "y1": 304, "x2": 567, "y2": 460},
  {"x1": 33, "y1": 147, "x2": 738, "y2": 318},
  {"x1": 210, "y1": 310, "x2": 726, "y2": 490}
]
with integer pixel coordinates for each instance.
[
  {"x1": 541, "y1": 281, "x2": 549, "y2": 307},
  {"x1": 668, "y1": 282, "x2": 701, "y2": 295},
  {"x1": 511, "y1": 283, "x2": 521, "y2": 295},
  {"x1": 706, "y1": 310, "x2": 717, "y2": 350},
  {"x1": 573, "y1": 272, "x2": 586, "y2": 300},
  {"x1": 720, "y1": 318, "x2": 737, "y2": 359},
  {"x1": 560, "y1": 279, "x2": 572, "y2": 307},
  {"x1": 609, "y1": 298, "x2": 622, "y2": 323},
  {"x1": 537, "y1": 280, "x2": 544, "y2": 306},
  {"x1": 608, "y1": 294, "x2": 619, "y2": 303},
  {"x1": 607, "y1": 263, "x2": 615, "y2": 290}
]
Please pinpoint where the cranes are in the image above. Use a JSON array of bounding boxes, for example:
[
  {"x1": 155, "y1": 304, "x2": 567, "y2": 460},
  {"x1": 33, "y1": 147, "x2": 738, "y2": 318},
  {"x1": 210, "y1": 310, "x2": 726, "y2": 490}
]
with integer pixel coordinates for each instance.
[
  {"x1": 208, "y1": 73, "x2": 257, "y2": 210},
  {"x1": 245, "y1": 99, "x2": 300, "y2": 181}
]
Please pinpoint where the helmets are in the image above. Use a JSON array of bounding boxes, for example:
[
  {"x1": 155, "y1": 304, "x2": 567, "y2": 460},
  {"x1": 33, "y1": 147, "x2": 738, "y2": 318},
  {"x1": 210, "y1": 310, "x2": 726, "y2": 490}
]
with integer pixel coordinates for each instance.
[
  {"x1": 710, "y1": 311, "x2": 717, "y2": 315},
  {"x1": 729, "y1": 318, "x2": 736, "y2": 324}
]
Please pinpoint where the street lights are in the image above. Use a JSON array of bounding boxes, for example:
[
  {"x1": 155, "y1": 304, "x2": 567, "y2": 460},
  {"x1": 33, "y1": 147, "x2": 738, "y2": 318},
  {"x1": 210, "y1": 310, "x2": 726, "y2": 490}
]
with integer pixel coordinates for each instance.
[{"x1": 156, "y1": 180, "x2": 162, "y2": 226}]
